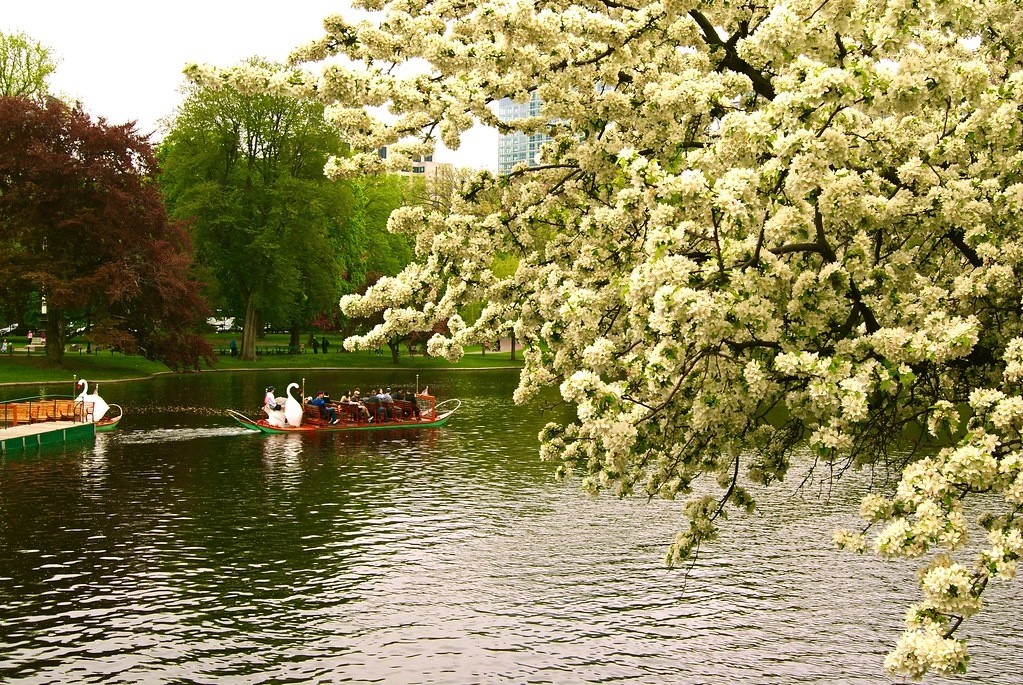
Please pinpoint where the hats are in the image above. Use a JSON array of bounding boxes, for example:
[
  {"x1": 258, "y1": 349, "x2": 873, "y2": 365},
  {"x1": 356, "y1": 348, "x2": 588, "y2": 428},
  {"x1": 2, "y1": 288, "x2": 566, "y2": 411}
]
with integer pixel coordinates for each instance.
[
  {"x1": 386, "y1": 388, "x2": 391, "y2": 391},
  {"x1": 268, "y1": 386, "x2": 276, "y2": 391}
]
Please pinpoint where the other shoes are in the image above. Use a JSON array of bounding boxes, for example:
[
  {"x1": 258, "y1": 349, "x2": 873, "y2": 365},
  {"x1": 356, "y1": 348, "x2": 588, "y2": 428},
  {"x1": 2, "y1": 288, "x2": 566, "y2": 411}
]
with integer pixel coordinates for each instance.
[
  {"x1": 328, "y1": 418, "x2": 332, "y2": 424},
  {"x1": 333, "y1": 419, "x2": 340, "y2": 424},
  {"x1": 368, "y1": 416, "x2": 374, "y2": 423}
]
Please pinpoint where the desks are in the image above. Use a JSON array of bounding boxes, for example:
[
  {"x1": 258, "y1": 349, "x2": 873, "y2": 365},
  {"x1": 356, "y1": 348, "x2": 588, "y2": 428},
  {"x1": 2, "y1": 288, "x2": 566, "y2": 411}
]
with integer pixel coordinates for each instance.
[{"x1": 26, "y1": 345, "x2": 46, "y2": 356}]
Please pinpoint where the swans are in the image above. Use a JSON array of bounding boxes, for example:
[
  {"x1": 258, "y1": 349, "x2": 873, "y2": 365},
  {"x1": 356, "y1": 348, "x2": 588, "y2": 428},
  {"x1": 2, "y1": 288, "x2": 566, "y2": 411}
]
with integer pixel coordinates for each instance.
[
  {"x1": 74, "y1": 379, "x2": 111, "y2": 420},
  {"x1": 284, "y1": 383, "x2": 303, "y2": 427}
]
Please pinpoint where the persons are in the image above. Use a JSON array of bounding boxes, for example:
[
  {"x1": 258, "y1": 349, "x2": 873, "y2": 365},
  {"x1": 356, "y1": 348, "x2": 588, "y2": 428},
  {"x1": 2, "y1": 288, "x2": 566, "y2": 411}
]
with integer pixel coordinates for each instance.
[
  {"x1": 496, "y1": 338, "x2": 500, "y2": 350},
  {"x1": 481, "y1": 343, "x2": 485, "y2": 356},
  {"x1": 340, "y1": 386, "x2": 401, "y2": 423},
  {"x1": 312, "y1": 337, "x2": 318, "y2": 354},
  {"x1": 229, "y1": 337, "x2": 237, "y2": 358},
  {"x1": 1, "y1": 338, "x2": 8, "y2": 354},
  {"x1": 40, "y1": 332, "x2": 46, "y2": 344},
  {"x1": 397, "y1": 389, "x2": 410, "y2": 417},
  {"x1": 264, "y1": 385, "x2": 281, "y2": 411},
  {"x1": 322, "y1": 337, "x2": 327, "y2": 354},
  {"x1": 304, "y1": 391, "x2": 340, "y2": 424},
  {"x1": 27, "y1": 330, "x2": 33, "y2": 345},
  {"x1": 404, "y1": 390, "x2": 422, "y2": 421}
]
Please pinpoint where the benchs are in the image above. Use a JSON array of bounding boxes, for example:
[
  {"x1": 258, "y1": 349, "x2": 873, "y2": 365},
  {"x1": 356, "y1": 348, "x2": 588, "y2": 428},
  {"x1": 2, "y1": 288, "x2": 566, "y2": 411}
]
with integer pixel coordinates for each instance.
[
  {"x1": 393, "y1": 400, "x2": 420, "y2": 420},
  {"x1": 255, "y1": 346, "x2": 301, "y2": 356},
  {"x1": 0, "y1": 399, "x2": 95, "y2": 424},
  {"x1": 213, "y1": 345, "x2": 242, "y2": 356},
  {"x1": 301, "y1": 403, "x2": 330, "y2": 428},
  {"x1": 381, "y1": 401, "x2": 404, "y2": 422},
  {"x1": 364, "y1": 402, "x2": 386, "y2": 423},
  {"x1": 325, "y1": 404, "x2": 350, "y2": 427},
  {"x1": 330, "y1": 400, "x2": 366, "y2": 424}
]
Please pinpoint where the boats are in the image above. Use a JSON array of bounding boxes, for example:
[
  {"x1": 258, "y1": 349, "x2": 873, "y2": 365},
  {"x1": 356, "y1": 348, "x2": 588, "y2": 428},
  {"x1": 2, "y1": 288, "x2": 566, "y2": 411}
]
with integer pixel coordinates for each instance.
[
  {"x1": 0, "y1": 373, "x2": 122, "y2": 455},
  {"x1": 226, "y1": 374, "x2": 461, "y2": 434}
]
